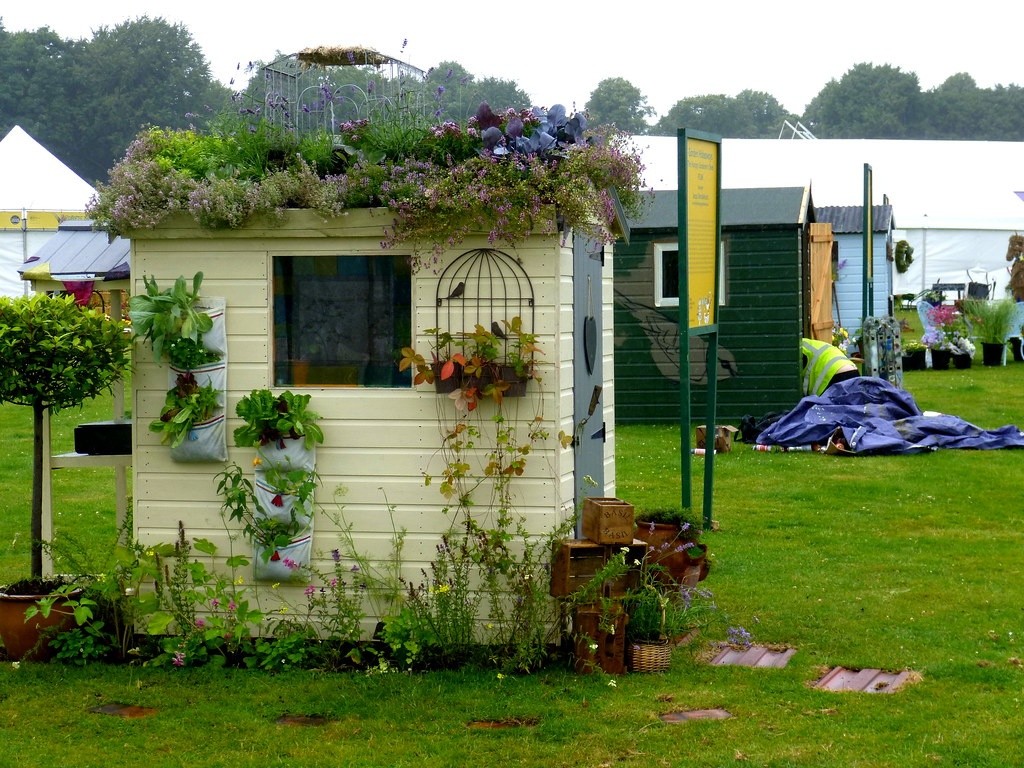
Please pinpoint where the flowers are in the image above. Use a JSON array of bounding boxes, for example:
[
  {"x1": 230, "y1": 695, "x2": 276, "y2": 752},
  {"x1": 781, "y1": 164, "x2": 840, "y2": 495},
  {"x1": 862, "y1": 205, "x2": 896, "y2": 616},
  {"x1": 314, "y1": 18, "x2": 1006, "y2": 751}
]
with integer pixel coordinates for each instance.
[
  {"x1": 832, "y1": 259, "x2": 847, "y2": 281},
  {"x1": 921, "y1": 329, "x2": 947, "y2": 350},
  {"x1": 831, "y1": 323, "x2": 850, "y2": 354},
  {"x1": 926, "y1": 304, "x2": 958, "y2": 325},
  {"x1": 948, "y1": 331, "x2": 978, "y2": 359}
]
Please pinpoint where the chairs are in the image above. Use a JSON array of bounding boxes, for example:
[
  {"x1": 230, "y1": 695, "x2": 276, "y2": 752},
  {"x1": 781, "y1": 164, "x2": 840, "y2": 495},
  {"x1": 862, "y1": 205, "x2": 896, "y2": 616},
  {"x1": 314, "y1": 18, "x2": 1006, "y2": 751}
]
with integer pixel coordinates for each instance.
[
  {"x1": 1003, "y1": 301, "x2": 1024, "y2": 365},
  {"x1": 917, "y1": 302, "x2": 959, "y2": 335}
]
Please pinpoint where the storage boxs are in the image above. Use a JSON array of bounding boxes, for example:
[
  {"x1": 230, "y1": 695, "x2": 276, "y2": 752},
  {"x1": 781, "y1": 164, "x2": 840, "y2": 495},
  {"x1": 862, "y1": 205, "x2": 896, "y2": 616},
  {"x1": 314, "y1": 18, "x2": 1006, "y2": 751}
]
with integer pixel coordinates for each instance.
[
  {"x1": 550, "y1": 496, "x2": 652, "y2": 675},
  {"x1": 696, "y1": 425, "x2": 739, "y2": 453}
]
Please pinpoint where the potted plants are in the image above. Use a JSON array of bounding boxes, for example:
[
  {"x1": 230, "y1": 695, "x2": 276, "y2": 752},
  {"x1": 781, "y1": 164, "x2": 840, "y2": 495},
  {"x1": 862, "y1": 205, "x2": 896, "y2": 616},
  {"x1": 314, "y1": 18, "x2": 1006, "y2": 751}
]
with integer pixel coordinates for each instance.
[
  {"x1": 902, "y1": 341, "x2": 926, "y2": 371},
  {"x1": 0, "y1": 287, "x2": 137, "y2": 662},
  {"x1": 963, "y1": 294, "x2": 1017, "y2": 366},
  {"x1": 398, "y1": 316, "x2": 578, "y2": 552},
  {"x1": 634, "y1": 507, "x2": 704, "y2": 600}
]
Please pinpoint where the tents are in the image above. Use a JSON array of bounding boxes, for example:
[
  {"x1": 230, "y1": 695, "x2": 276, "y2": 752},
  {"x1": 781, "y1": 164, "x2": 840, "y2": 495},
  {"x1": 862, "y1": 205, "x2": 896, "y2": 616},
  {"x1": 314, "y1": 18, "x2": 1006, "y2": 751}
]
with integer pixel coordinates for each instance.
[
  {"x1": 610, "y1": 135, "x2": 1024, "y2": 305},
  {"x1": 0, "y1": 125, "x2": 102, "y2": 299}
]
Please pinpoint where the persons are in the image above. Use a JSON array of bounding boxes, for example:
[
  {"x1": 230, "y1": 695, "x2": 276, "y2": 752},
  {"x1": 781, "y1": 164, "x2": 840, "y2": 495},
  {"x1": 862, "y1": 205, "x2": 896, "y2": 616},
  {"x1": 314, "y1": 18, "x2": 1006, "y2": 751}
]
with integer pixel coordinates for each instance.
[{"x1": 802, "y1": 338, "x2": 861, "y2": 397}]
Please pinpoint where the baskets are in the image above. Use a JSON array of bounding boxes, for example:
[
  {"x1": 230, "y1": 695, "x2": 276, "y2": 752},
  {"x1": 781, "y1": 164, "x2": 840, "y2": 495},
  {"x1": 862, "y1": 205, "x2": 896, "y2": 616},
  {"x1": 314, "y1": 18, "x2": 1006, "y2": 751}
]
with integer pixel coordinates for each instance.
[{"x1": 625, "y1": 585, "x2": 672, "y2": 675}]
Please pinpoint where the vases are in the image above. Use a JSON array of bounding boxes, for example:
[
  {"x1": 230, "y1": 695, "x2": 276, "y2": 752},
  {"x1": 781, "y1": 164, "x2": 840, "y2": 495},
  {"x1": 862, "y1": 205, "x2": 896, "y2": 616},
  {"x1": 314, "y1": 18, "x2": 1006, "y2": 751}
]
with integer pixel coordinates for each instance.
[
  {"x1": 932, "y1": 349, "x2": 951, "y2": 370},
  {"x1": 951, "y1": 353, "x2": 971, "y2": 369}
]
